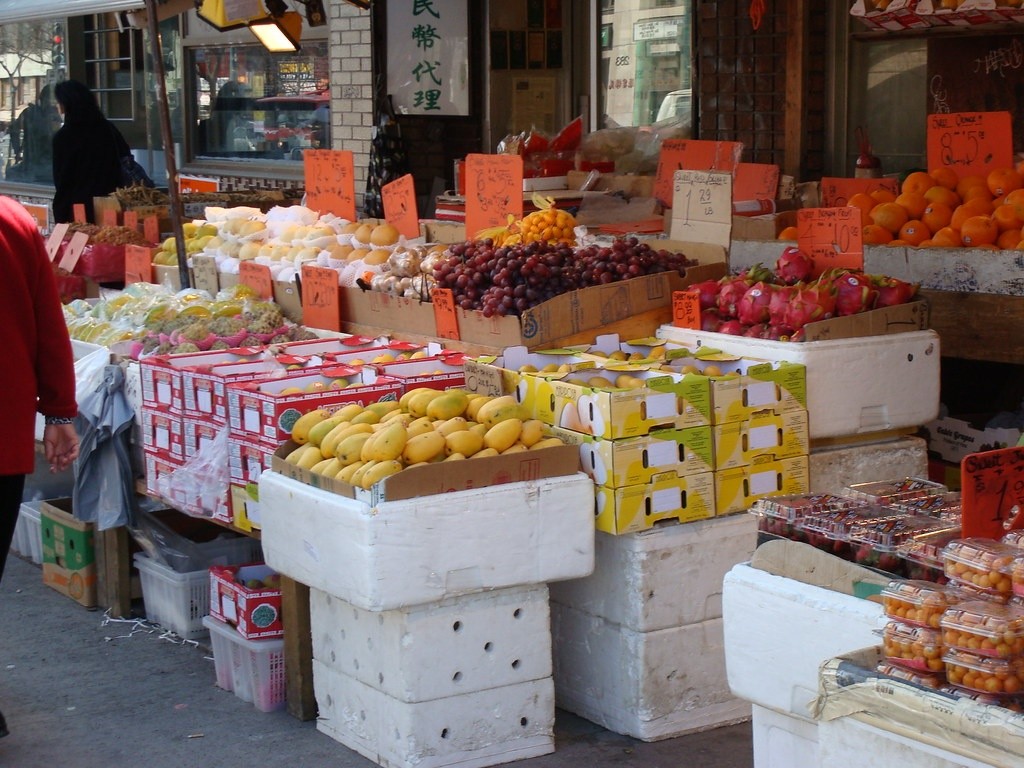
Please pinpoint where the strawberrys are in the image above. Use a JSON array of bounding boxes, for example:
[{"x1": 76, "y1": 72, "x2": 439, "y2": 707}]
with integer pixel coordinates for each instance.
[{"x1": 757, "y1": 480, "x2": 960, "y2": 584}]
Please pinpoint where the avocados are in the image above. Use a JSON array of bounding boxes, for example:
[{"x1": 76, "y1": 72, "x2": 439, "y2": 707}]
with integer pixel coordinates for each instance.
[{"x1": 153, "y1": 217, "x2": 399, "y2": 287}]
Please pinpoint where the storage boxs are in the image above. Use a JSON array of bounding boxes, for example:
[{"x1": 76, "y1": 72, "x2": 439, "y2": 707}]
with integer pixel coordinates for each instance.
[{"x1": 14, "y1": 169, "x2": 1024, "y2": 767}]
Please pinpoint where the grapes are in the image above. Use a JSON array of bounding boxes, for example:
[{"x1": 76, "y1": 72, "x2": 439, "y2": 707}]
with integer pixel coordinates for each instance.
[{"x1": 435, "y1": 236, "x2": 698, "y2": 317}]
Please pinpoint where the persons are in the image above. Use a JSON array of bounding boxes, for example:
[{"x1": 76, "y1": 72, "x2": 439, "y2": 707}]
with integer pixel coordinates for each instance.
[
  {"x1": 1, "y1": 195, "x2": 80, "y2": 583},
  {"x1": 52, "y1": 81, "x2": 132, "y2": 226},
  {"x1": 11, "y1": 85, "x2": 61, "y2": 160},
  {"x1": 196, "y1": 82, "x2": 280, "y2": 156}
]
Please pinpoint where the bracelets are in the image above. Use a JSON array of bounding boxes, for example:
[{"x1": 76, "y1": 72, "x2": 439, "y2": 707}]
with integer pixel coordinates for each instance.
[{"x1": 43, "y1": 414, "x2": 74, "y2": 425}]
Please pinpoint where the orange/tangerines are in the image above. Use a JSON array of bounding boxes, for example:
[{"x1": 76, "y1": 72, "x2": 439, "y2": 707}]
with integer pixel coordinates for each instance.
[
  {"x1": 776, "y1": 167, "x2": 1023, "y2": 253},
  {"x1": 884, "y1": 535, "x2": 1024, "y2": 693}
]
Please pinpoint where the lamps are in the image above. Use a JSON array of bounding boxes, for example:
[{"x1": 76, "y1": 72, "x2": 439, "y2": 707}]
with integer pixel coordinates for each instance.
[
  {"x1": 250, "y1": 1, "x2": 305, "y2": 55},
  {"x1": 194, "y1": 0, "x2": 272, "y2": 34}
]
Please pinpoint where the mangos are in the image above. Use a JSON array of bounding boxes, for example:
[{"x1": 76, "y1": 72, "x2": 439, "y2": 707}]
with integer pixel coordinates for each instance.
[
  {"x1": 523, "y1": 345, "x2": 737, "y2": 389},
  {"x1": 283, "y1": 384, "x2": 568, "y2": 486}
]
